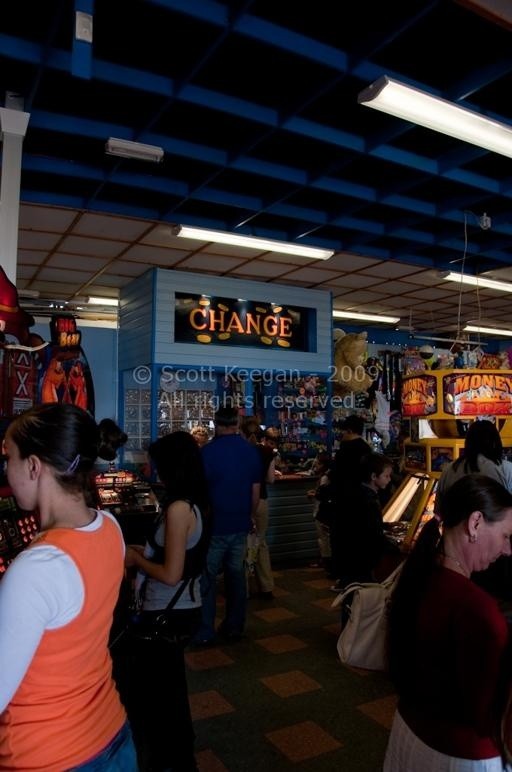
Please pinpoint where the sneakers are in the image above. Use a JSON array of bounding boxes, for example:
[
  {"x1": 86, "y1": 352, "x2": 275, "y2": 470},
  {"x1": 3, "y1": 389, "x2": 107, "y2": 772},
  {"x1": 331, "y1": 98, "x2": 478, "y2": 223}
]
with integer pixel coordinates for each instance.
[
  {"x1": 251, "y1": 591, "x2": 273, "y2": 600},
  {"x1": 312, "y1": 560, "x2": 330, "y2": 568},
  {"x1": 185, "y1": 621, "x2": 242, "y2": 649},
  {"x1": 330, "y1": 579, "x2": 345, "y2": 592}
]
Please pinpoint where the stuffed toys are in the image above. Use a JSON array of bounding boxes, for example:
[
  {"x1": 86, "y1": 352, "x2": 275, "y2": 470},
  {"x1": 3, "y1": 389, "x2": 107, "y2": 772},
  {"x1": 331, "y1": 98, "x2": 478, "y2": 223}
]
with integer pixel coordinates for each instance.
[{"x1": 333, "y1": 329, "x2": 374, "y2": 399}]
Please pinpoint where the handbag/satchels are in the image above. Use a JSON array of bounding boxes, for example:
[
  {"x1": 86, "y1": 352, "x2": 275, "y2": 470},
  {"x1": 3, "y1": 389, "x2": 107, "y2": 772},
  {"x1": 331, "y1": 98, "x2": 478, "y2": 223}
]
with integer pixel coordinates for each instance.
[
  {"x1": 109, "y1": 613, "x2": 167, "y2": 660},
  {"x1": 336, "y1": 584, "x2": 396, "y2": 672}
]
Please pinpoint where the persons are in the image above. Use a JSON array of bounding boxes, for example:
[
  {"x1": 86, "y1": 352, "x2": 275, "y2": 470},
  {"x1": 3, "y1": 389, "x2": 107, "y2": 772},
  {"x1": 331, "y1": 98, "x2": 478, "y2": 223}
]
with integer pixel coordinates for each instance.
[
  {"x1": 305, "y1": 415, "x2": 512, "y2": 772},
  {"x1": 0, "y1": 404, "x2": 287, "y2": 772}
]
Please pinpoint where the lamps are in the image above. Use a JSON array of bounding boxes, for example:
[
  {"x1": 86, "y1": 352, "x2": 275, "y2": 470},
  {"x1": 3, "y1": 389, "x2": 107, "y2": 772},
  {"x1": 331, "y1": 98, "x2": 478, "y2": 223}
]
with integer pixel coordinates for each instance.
[
  {"x1": 332, "y1": 310, "x2": 400, "y2": 325},
  {"x1": 172, "y1": 225, "x2": 336, "y2": 260},
  {"x1": 439, "y1": 271, "x2": 512, "y2": 293},
  {"x1": 87, "y1": 296, "x2": 119, "y2": 307},
  {"x1": 462, "y1": 325, "x2": 512, "y2": 336},
  {"x1": 357, "y1": 75, "x2": 512, "y2": 160}
]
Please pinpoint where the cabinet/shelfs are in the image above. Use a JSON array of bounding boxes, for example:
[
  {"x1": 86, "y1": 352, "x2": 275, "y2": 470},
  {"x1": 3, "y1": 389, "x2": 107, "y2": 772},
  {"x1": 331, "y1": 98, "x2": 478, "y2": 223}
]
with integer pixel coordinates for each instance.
[{"x1": 124, "y1": 388, "x2": 215, "y2": 449}]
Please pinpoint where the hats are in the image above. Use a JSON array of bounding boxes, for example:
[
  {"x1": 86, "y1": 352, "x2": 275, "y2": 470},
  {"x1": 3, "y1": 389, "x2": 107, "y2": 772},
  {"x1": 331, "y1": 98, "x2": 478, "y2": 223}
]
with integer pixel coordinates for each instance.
[{"x1": 332, "y1": 416, "x2": 362, "y2": 436}]
